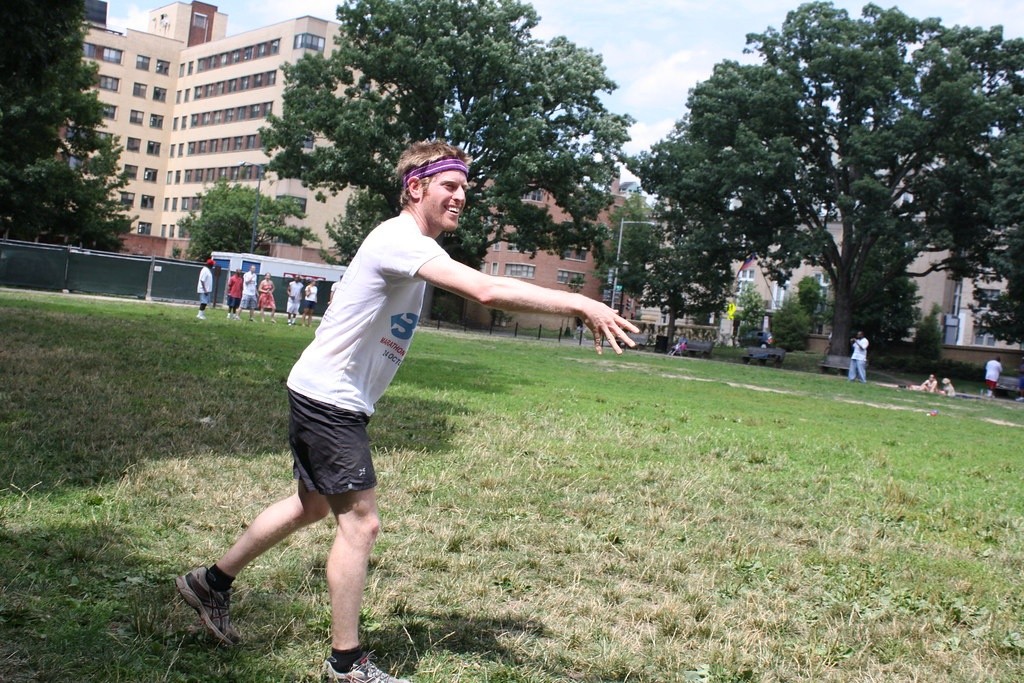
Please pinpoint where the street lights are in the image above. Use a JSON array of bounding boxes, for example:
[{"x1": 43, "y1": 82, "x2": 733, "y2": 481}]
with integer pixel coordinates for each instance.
[
  {"x1": 237, "y1": 161, "x2": 264, "y2": 255},
  {"x1": 611, "y1": 217, "x2": 656, "y2": 308}
]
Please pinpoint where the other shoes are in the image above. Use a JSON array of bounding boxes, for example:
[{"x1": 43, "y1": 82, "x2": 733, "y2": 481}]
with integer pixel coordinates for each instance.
[
  {"x1": 271, "y1": 318, "x2": 277, "y2": 323},
  {"x1": 227, "y1": 314, "x2": 231, "y2": 318},
  {"x1": 236, "y1": 316, "x2": 241, "y2": 320},
  {"x1": 249, "y1": 318, "x2": 256, "y2": 323},
  {"x1": 288, "y1": 320, "x2": 296, "y2": 326},
  {"x1": 196, "y1": 315, "x2": 207, "y2": 320},
  {"x1": 233, "y1": 315, "x2": 236, "y2": 320},
  {"x1": 227, "y1": 315, "x2": 230, "y2": 318}
]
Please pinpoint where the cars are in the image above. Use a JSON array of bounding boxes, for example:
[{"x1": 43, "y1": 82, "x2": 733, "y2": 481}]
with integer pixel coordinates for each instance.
[{"x1": 739, "y1": 331, "x2": 772, "y2": 349}]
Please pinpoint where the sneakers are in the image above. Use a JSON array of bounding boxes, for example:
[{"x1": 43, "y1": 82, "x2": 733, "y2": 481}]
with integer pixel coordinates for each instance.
[
  {"x1": 175, "y1": 565, "x2": 243, "y2": 645},
  {"x1": 320, "y1": 655, "x2": 411, "y2": 683}
]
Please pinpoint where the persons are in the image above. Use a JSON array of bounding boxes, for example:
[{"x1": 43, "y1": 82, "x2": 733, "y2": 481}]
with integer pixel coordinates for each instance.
[
  {"x1": 984, "y1": 355, "x2": 1003, "y2": 398},
  {"x1": 286, "y1": 274, "x2": 304, "y2": 327},
  {"x1": 1013, "y1": 357, "x2": 1024, "y2": 402},
  {"x1": 916, "y1": 374, "x2": 937, "y2": 392},
  {"x1": 235, "y1": 265, "x2": 259, "y2": 321},
  {"x1": 196, "y1": 259, "x2": 216, "y2": 320},
  {"x1": 227, "y1": 268, "x2": 243, "y2": 320},
  {"x1": 302, "y1": 279, "x2": 319, "y2": 328},
  {"x1": 848, "y1": 331, "x2": 869, "y2": 384},
  {"x1": 573, "y1": 316, "x2": 586, "y2": 340},
  {"x1": 941, "y1": 378, "x2": 955, "y2": 397},
  {"x1": 175, "y1": 138, "x2": 641, "y2": 683},
  {"x1": 258, "y1": 273, "x2": 276, "y2": 323},
  {"x1": 327, "y1": 275, "x2": 343, "y2": 305}
]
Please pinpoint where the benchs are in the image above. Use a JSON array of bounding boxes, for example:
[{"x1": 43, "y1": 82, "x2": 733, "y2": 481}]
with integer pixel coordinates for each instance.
[
  {"x1": 674, "y1": 339, "x2": 714, "y2": 357},
  {"x1": 816, "y1": 354, "x2": 869, "y2": 376},
  {"x1": 616, "y1": 333, "x2": 651, "y2": 352},
  {"x1": 993, "y1": 375, "x2": 1022, "y2": 399},
  {"x1": 740, "y1": 346, "x2": 786, "y2": 368}
]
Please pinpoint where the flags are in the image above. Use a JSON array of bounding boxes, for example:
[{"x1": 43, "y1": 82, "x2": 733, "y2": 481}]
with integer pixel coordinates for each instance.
[{"x1": 736, "y1": 251, "x2": 756, "y2": 276}]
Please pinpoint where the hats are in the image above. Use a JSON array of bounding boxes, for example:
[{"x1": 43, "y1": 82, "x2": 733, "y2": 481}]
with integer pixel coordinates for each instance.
[{"x1": 207, "y1": 259, "x2": 216, "y2": 265}]
